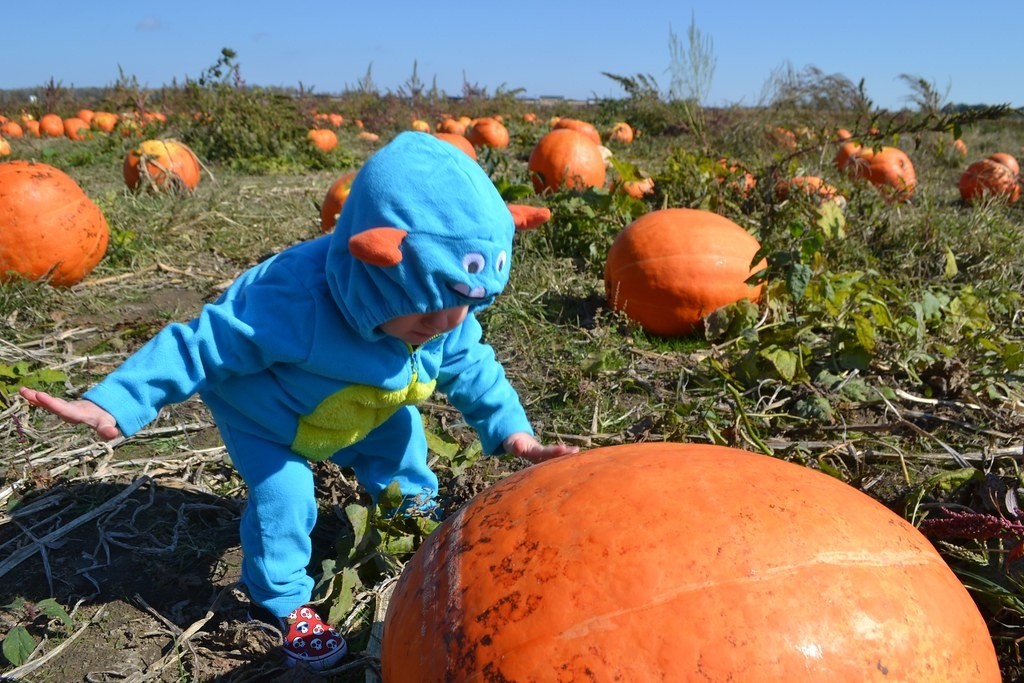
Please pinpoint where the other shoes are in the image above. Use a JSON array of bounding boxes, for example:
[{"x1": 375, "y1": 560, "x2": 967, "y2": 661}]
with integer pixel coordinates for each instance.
[{"x1": 245, "y1": 604, "x2": 347, "y2": 671}]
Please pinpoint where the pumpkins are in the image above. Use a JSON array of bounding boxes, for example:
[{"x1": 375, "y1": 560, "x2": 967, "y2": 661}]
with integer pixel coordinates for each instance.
[{"x1": 0, "y1": 109, "x2": 1022, "y2": 682}]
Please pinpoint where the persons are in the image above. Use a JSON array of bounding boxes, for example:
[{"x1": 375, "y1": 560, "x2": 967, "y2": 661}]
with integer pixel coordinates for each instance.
[{"x1": 19, "y1": 130, "x2": 581, "y2": 670}]
